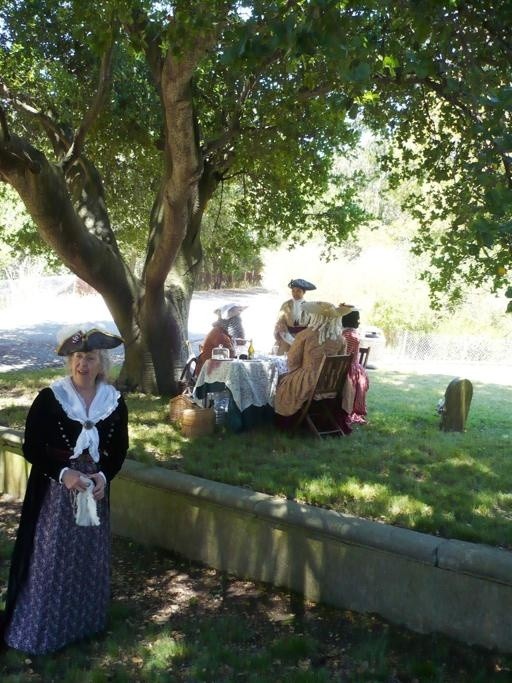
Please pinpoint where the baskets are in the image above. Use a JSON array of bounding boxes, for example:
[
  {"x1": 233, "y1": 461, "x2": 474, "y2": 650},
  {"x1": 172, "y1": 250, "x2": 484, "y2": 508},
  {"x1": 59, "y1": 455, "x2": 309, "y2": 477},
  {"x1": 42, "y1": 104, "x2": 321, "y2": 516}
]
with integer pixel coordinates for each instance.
[{"x1": 169, "y1": 393, "x2": 215, "y2": 436}]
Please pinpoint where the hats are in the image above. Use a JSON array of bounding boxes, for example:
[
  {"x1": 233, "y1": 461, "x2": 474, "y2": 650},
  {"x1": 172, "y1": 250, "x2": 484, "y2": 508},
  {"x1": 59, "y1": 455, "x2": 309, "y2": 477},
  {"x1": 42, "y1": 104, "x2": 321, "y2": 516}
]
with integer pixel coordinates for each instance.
[
  {"x1": 289, "y1": 279, "x2": 317, "y2": 289},
  {"x1": 56, "y1": 329, "x2": 125, "y2": 356},
  {"x1": 221, "y1": 304, "x2": 248, "y2": 319},
  {"x1": 300, "y1": 301, "x2": 352, "y2": 319}
]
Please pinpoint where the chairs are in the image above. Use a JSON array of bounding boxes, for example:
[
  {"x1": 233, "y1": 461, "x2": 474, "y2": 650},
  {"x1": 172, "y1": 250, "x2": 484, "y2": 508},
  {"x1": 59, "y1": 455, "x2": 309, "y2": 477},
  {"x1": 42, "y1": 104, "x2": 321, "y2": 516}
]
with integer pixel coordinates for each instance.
[
  {"x1": 181, "y1": 355, "x2": 201, "y2": 400},
  {"x1": 357, "y1": 346, "x2": 372, "y2": 372},
  {"x1": 286, "y1": 351, "x2": 356, "y2": 443}
]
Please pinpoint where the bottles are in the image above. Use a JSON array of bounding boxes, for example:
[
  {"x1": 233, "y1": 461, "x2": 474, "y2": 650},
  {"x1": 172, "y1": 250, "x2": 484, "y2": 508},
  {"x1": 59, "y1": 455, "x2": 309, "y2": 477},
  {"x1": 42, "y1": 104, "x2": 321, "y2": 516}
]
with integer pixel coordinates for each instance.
[{"x1": 247, "y1": 338, "x2": 255, "y2": 358}]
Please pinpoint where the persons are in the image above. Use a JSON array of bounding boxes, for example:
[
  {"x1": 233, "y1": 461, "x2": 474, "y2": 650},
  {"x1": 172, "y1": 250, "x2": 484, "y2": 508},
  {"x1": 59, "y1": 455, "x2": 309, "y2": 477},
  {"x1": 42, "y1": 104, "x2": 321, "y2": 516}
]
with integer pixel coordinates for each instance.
[
  {"x1": 195, "y1": 278, "x2": 370, "y2": 431},
  {"x1": 3, "y1": 327, "x2": 128, "y2": 655}
]
